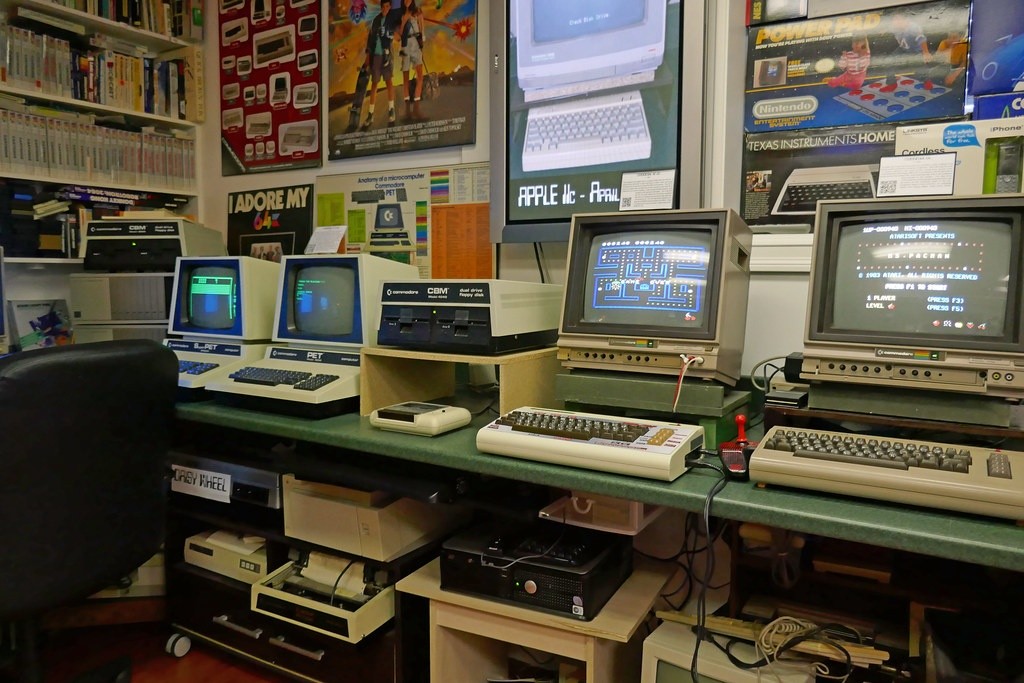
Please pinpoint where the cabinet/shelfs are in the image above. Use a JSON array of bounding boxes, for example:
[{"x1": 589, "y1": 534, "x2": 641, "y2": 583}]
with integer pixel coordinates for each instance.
[
  {"x1": 167, "y1": 500, "x2": 439, "y2": 683},
  {"x1": 0, "y1": 0, "x2": 205, "y2": 265}
]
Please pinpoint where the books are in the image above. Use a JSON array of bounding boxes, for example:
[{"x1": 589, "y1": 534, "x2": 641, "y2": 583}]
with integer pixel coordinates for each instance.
[
  {"x1": 11, "y1": 185, "x2": 91, "y2": 259},
  {"x1": 100, "y1": 208, "x2": 185, "y2": 219},
  {"x1": 0, "y1": 0, "x2": 205, "y2": 193}
]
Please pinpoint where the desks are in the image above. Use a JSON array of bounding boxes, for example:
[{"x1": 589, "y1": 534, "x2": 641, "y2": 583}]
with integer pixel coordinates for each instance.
[
  {"x1": 396, "y1": 554, "x2": 680, "y2": 683},
  {"x1": 172, "y1": 403, "x2": 1024, "y2": 683}
]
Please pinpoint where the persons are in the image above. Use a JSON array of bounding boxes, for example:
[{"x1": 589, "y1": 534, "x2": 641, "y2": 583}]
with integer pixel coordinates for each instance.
[
  {"x1": 361, "y1": 0, "x2": 429, "y2": 128},
  {"x1": 822, "y1": 7, "x2": 933, "y2": 94},
  {"x1": 748, "y1": 173, "x2": 767, "y2": 191},
  {"x1": 251, "y1": 245, "x2": 282, "y2": 262}
]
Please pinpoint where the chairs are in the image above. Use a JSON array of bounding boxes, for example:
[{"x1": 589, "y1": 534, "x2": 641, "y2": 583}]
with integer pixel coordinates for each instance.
[{"x1": 0, "y1": 338, "x2": 180, "y2": 683}]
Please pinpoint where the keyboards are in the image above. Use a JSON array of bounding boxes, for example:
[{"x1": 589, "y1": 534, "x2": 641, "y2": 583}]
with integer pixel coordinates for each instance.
[
  {"x1": 781, "y1": 181, "x2": 873, "y2": 210},
  {"x1": 176, "y1": 359, "x2": 219, "y2": 375},
  {"x1": 521, "y1": 90, "x2": 652, "y2": 172},
  {"x1": 475, "y1": 405, "x2": 705, "y2": 481},
  {"x1": 749, "y1": 425, "x2": 1024, "y2": 520},
  {"x1": 655, "y1": 610, "x2": 890, "y2": 660},
  {"x1": 228, "y1": 366, "x2": 339, "y2": 391}
]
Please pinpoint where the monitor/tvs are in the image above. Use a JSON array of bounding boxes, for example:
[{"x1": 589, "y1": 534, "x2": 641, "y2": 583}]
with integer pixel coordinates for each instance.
[
  {"x1": 273, "y1": 255, "x2": 418, "y2": 353},
  {"x1": 800, "y1": 197, "x2": 1024, "y2": 427},
  {"x1": 556, "y1": 207, "x2": 753, "y2": 387},
  {"x1": 509, "y1": 0, "x2": 667, "y2": 101},
  {"x1": 168, "y1": 256, "x2": 281, "y2": 345}
]
